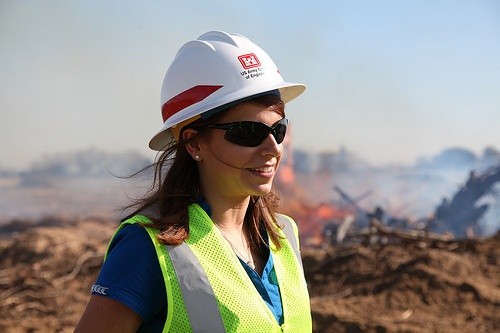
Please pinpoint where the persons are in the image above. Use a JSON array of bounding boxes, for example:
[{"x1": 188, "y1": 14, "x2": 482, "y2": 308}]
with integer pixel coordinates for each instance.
[{"x1": 69, "y1": 26, "x2": 313, "y2": 333}]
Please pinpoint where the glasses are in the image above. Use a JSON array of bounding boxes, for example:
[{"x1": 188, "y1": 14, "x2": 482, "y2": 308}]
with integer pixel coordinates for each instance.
[{"x1": 201, "y1": 118, "x2": 289, "y2": 148}]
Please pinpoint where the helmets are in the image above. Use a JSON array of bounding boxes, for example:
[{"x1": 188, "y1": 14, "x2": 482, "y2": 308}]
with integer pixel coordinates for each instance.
[{"x1": 149, "y1": 30, "x2": 307, "y2": 152}]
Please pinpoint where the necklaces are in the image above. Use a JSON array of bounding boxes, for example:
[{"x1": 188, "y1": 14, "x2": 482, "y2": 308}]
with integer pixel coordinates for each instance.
[{"x1": 214, "y1": 221, "x2": 255, "y2": 269}]
